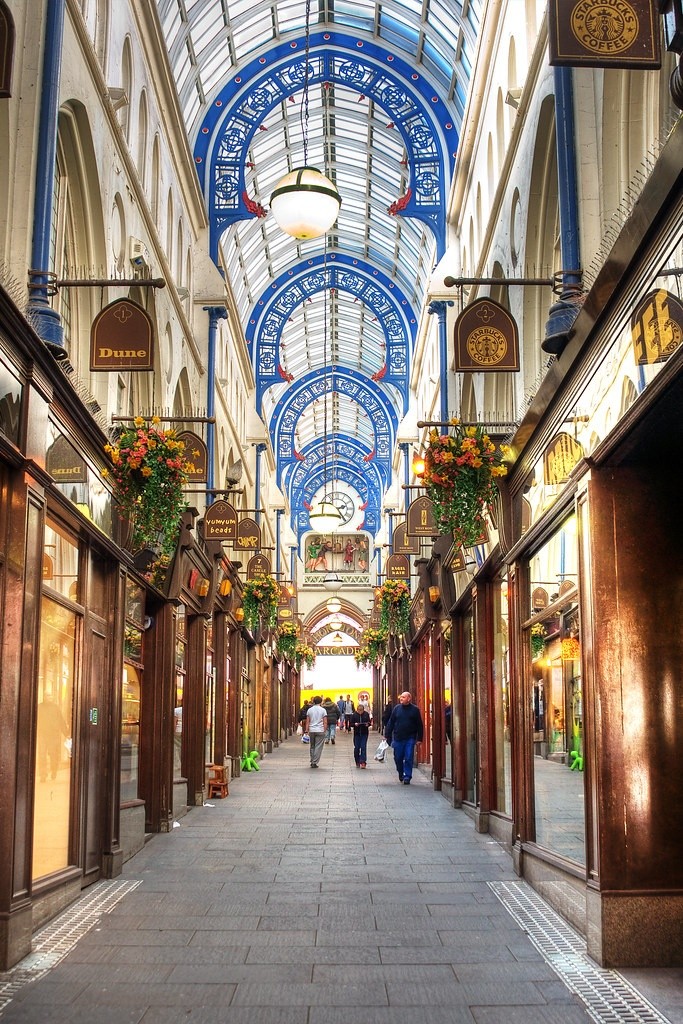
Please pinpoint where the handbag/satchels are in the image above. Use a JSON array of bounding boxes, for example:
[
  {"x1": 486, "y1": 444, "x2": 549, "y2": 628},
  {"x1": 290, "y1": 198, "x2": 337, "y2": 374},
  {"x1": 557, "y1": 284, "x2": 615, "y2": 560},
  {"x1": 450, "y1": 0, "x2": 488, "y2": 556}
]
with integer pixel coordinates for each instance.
[
  {"x1": 373, "y1": 740, "x2": 389, "y2": 764},
  {"x1": 296, "y1": 723, "x2": 303, "y2": 736},
  {"x1": 301, "y1": 734, "x2": 310, "y2": 744}
]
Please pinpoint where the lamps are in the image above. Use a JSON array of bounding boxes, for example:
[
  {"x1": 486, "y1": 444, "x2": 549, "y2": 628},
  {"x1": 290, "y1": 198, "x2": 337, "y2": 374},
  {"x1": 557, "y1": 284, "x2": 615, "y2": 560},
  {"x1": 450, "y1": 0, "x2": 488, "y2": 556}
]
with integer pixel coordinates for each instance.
[
  {"x1": 308, "y1": 233, "x2": 341, "y2": 533},
  {"x1": 222, "y1": 459, "x2": 243, "y2": 501},
  {"x1": 558, "y1": 630, "x2": 581, "y2": 659},
  {"x1": 323, "y1": 286, "x2": 344, "y2": 591},
  {"x1": 278, "y1": 580, "x2": 295, "y2": 596},
  {"x1": 270, "y1": 0, "x2": 343, "y2": 242},
  {"x1": 412, "y1": 450, "x2": 427, "y2": 479},
  {"x1": 325, "y1": 590, "x2": 344, "y2": 644}
]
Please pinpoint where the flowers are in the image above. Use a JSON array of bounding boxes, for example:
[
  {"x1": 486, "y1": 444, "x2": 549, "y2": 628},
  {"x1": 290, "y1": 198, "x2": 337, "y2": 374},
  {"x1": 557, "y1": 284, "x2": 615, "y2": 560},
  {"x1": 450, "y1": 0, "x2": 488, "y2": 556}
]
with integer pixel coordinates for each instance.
[
  {"x1": 423, "y1": 429, "x2": 509, "y2": 554},
  {"x1": 124, "y1": 628, "x2": 139, "y2": 660},
  {"x1": 239, "y1": 573, "x2": 283, "y2": 632},
  {"x1": 100, "y1": 414, "x2": 193, "y2": 558},
  {"x1": 353, "y1": 628, "x2": 388, "y2": 671},
  {"x1": 531, "y1": 623, "x2": 546, "y2": 657},
  {"x1": 276, "y1": 620, "x2": 301, "y2": 660},
  {"x1": 295, "y1": 644, "x2": 317, "y2": 671},
  {"x1": 379, "y1": 578, "x2": 411, "y2": 634}
]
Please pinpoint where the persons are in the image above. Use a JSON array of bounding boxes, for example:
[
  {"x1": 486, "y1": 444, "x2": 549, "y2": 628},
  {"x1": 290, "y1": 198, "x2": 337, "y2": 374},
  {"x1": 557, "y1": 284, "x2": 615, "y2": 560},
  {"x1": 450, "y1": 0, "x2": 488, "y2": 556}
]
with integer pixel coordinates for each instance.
[
  {"x1": 337, "y1": 696, "x2": 345, "y2": 730},
  {"x1": 298, "y1": 697, "x2": 314, "y2": 743},
  {"x1": 322, "y1": 697, "x2": 340, "y2": 744},
  {"x1": 344, "y1": 695, "x2": 355, "y2": 733},
  {"x1": 385, "y1": 691, "x2": 423, "y2": 784},
  {"x1": 173, "y1": 701, "x2": 182, "y2": 766},
  {"x1": 443, "y1": 700, "x2": 451, "y2": 746},
  {"x1": 305, "y1": 696, "x2": 327, "y2": 768},
  {"x1": 349, "y1": 705, "x2": 371, "y2": 768},
  {"x1": 358, "y1": 695, "x2": 371, "y2": 713},
  {"x1": 381, "y1": 695, "x2": 394, "y2": 727}
]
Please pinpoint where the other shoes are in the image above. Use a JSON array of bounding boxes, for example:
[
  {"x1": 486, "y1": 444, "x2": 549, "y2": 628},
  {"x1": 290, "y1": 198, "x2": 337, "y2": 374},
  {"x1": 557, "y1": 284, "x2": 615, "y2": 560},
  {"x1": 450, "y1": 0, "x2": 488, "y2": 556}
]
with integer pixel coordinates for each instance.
[
  {"x1": 310, "y1": 763, "x2": 318, "y2": 768},
  {"x1": 356, "y1": 762, "x2": 360, "y2": 768},
  {"x1": 325, "y1": 741, "x2": 329, "y2": 744},
  {"x1": 399, "y1": 772, "x2": 404, "y2": 782},
  {"x1": 331, "y1": 739, "x2": 335, "y2": 744},
  {"x1": 404, "y1": 780, "x2": 410, "y2": 784},
  {"x1": 360, "y1": 762, "x2": 366, "y2": 769}
]
